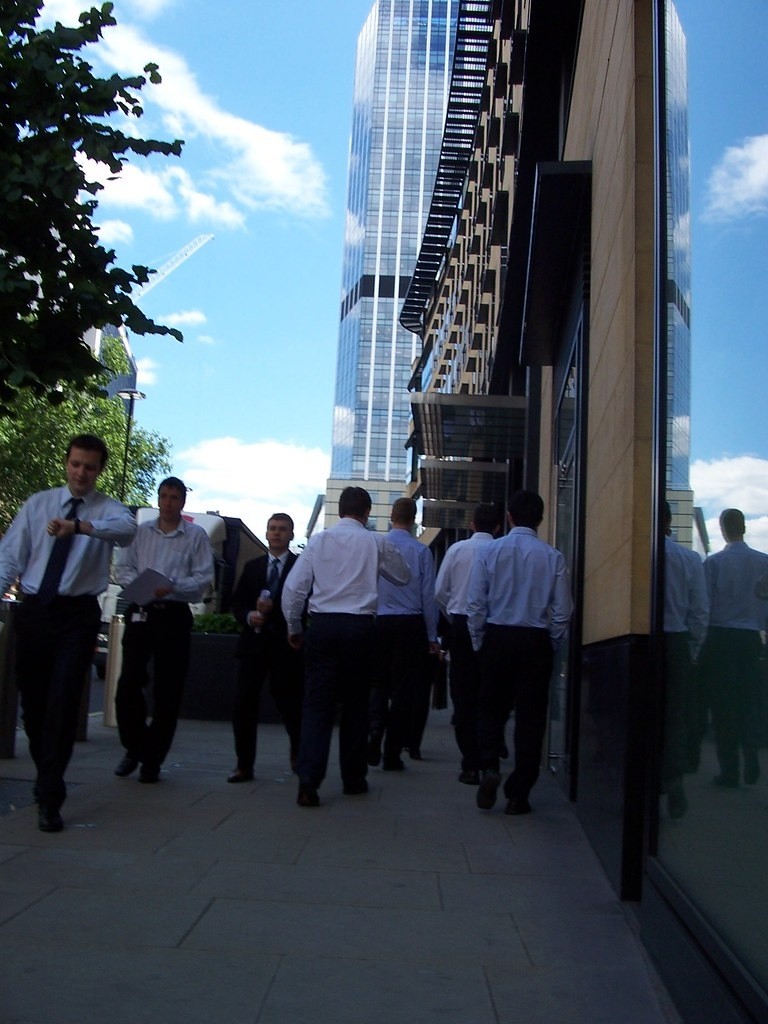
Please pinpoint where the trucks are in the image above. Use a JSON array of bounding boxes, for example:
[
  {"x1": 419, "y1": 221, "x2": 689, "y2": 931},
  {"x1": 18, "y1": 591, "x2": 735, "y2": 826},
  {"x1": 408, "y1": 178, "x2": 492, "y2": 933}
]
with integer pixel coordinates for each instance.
[{"x1": 95, "y1": 506, "x2": 269, "y2": 681}]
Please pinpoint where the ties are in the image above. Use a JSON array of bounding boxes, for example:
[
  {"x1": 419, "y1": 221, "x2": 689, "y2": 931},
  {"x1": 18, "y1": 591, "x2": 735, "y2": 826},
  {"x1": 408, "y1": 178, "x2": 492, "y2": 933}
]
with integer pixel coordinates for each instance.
[
  {"x1": 37, "y1": 498, "x2": 84, "y2": 608},
  {"x1": 264, "y1": 558, "x2": 281, "y2": 600}
]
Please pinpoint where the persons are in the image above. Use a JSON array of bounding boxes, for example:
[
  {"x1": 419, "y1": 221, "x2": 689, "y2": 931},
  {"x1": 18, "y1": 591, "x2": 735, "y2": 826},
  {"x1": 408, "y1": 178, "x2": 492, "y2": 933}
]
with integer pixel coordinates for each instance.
[
  {"x1": 468, "y1": 490, "x2": 574, "y2": 815},
  {"x1": 226, "y1": 513, "x2": 305, "y2": 783},
  {"x1": 113, "y1": 477, "x2": 215, "y2": 784},
  {"x1": 663, "y1": 497, "x2": 767, "y2": 822},
  {"x1": 435, "y1": 504, "x2": 509, "y2": 785},
  {"x1": 0, "y1": 433, "x2": 138, "y2": 832},
  {"x1": 368, "y1": 497, "x2": 440, "y2": 770},
  {"x1": 281, "y1": 485, "x2": 412, "y2": 806}
]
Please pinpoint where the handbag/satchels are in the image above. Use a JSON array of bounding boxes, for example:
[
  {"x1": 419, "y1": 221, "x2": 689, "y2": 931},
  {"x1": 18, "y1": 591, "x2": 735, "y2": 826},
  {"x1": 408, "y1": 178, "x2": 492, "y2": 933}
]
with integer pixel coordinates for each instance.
[{"x1": 432, "y1": 656, "x2": 447, "y2": 711}]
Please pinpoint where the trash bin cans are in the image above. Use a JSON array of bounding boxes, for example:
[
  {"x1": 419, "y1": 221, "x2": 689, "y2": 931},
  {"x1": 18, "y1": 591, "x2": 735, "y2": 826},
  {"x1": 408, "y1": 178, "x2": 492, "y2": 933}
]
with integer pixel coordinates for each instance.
[{"x1": 0, "y1": 599, "x2": 22, "y2": 759}]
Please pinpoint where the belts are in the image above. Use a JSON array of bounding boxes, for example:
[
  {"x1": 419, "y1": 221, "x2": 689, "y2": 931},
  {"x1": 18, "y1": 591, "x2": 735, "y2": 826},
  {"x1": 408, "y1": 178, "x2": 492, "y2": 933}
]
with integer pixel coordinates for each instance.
[{"x1": 144, "y1": 600, "x2": 189, "y2": 610}]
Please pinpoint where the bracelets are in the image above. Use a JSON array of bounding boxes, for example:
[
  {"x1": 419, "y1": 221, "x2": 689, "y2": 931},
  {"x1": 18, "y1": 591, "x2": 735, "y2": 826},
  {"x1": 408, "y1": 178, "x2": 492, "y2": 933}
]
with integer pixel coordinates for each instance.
[{"x1": 74, "y1": 518, "x2": 82, "y2": 534}]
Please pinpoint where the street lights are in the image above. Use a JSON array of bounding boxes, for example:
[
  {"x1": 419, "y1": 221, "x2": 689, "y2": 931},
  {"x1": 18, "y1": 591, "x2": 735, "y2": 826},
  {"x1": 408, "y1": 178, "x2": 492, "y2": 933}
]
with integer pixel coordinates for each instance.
[{"x1": 115, "y1": 387, "x2": 147, "y2": 504}]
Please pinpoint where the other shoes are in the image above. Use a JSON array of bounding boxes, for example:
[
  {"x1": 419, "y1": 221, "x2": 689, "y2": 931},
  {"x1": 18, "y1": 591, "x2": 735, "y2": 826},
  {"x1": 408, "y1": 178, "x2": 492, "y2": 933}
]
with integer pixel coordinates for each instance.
[
  {"x1": 714, "y1": 773, "x2": 738, "y2": 788},
  {"x1": 343, "y1": 778, "x2": 369, "y2": 794},
  {"x1": 228, "y1": 768, "x2": 253, "y2": 782},
  {"x1": 368, "y1": 733, "x2": 382, "y2": 765},
  {"x1": 114, "y1": 756, "x2": 137, "y2": 777},
  {"x1": 406, "y1": 746, "x2": 421, "y2": 760},
  {"x1": 744, "y1": 748, "x2": 759, "y2": 784},
  {"x1": 666, "y1": 765, "x2": 687, "y2": 818},
  {"x1": 36, "y1": 807, "x2": 63, "y2": 833},
  {"x1": 383, "y1": 760, "x2": 404, "y2": 770},
  {"x1": 290, "y1": 747, "x2": 300, "y2": 770},
  {"x1": 298, "y1": 789, "x2": 319, "y2": 806},
  {"x1": 139, "y1": 765, "x2": 159, "y2": 783},
  {"x1": 505, "y1": 799, "x2": 531, "y2": 814},
  {"x1": 459, "y1": 768, "x2": 479, "y2": 785},
  {"x1": 476, "y1": 774, "x2": 501, "y2": 808}
]
